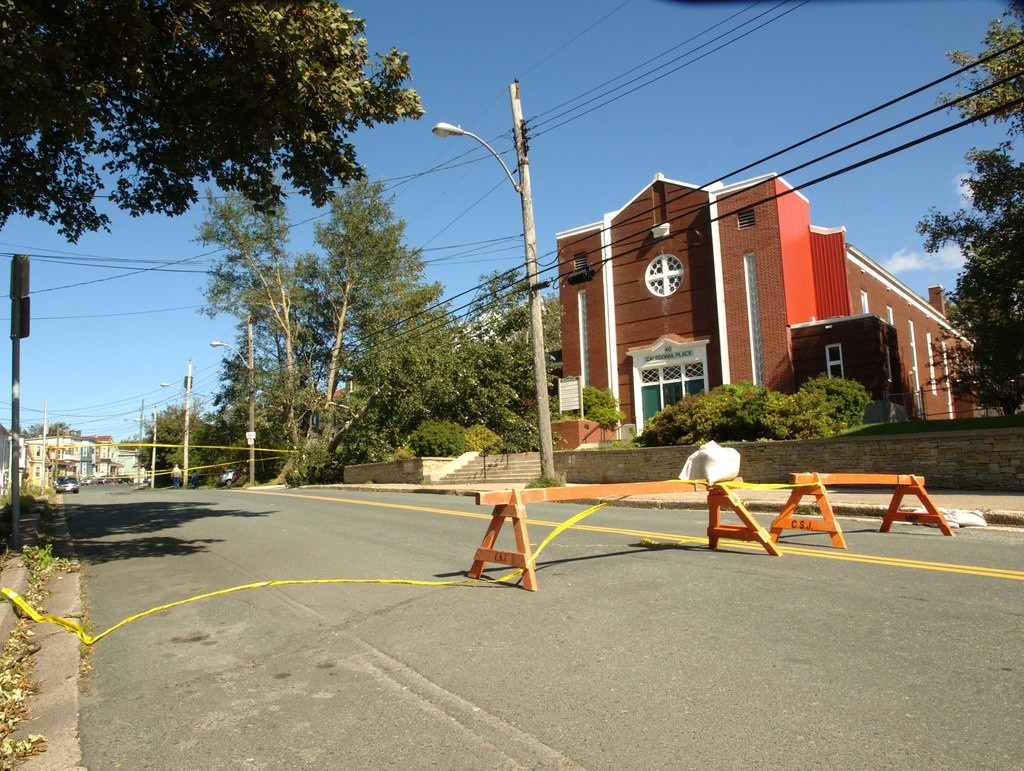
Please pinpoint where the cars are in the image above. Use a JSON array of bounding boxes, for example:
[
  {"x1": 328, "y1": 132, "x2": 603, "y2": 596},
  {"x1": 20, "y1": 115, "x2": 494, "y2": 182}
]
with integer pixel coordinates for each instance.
[
  {"x1": 79, "y1": 474, "x2": 130, "y2": 485},
  {"x1": 54, "y1": 476, "x2": 80, "y2": 494},
  {"x1": 219, "y1": 467, "x2": 238, "y2": 489}
]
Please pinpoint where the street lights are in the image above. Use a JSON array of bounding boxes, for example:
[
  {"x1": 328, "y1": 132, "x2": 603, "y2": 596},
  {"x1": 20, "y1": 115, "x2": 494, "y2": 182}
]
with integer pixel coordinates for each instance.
[
  {"x1": 432, "y1": 121, "x2": 555, "y2": 481},
  {"x1": 135, "y1": 418, "x2": 156, "y2": 488},
  {"x1": 211, "y1": 342, "x2": 255, "y2": 487},
  {"x1": 160, "y1": 383, "x2": 189, "y2": 487}
]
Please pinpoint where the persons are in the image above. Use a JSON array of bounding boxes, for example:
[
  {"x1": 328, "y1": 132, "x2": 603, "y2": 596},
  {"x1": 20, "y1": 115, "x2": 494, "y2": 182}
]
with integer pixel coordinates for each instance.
[
  {"x1": 171, "y1": 463, "x2": 181, "y2": 489},
  {"x1": 191, "y1": 470, "x2": 199, "y2": 490}
]
